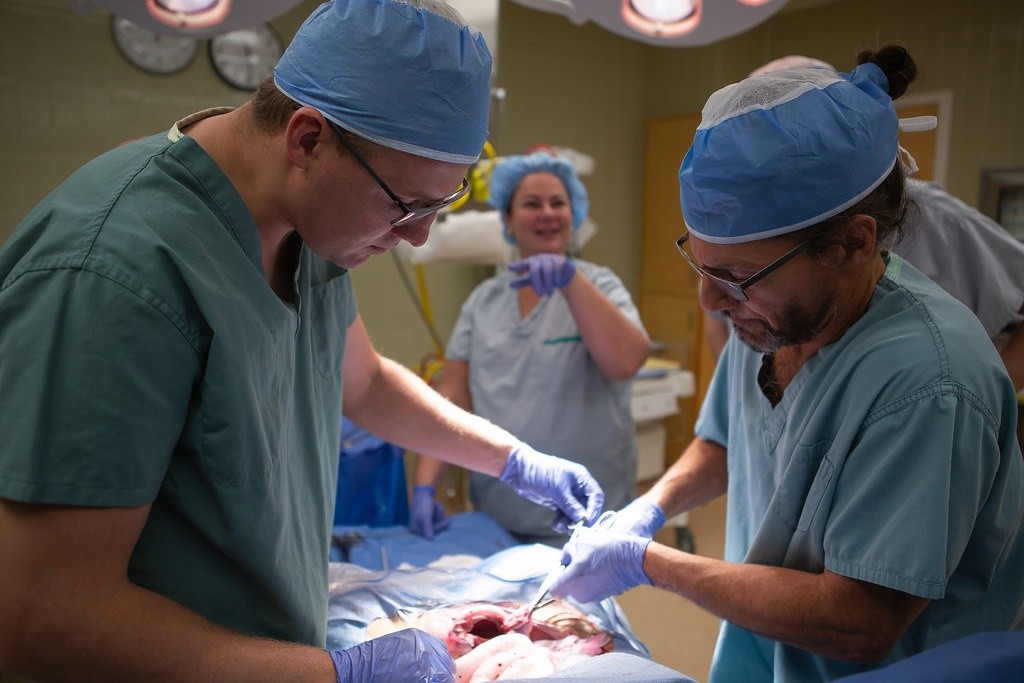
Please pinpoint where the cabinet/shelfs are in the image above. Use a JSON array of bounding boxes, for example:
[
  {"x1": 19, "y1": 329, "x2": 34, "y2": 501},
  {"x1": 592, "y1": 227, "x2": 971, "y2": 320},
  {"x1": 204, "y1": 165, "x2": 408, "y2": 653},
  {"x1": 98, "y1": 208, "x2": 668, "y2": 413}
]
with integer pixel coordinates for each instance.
[{"x1": 640, "y1": 117, "x2": 729, "y2": 466}]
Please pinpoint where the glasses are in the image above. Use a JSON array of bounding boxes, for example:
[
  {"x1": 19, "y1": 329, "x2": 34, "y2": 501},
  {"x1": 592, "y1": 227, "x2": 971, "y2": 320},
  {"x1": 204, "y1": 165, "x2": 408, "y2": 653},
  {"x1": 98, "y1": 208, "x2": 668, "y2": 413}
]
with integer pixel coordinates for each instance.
[
  {"x1": 674, "y1": 228, "x2": 842, "y2": 304},
  {"x1": 325, "y1": 116, "x2": 472, "y2": 229}
]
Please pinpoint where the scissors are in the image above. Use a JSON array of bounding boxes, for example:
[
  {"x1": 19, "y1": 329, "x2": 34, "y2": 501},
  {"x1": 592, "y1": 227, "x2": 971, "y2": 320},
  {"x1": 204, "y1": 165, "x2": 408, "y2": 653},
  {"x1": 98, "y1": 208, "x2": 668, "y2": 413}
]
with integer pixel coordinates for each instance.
[{"x1": 528, "y1": 510, "x2": 619, "y2": 617}]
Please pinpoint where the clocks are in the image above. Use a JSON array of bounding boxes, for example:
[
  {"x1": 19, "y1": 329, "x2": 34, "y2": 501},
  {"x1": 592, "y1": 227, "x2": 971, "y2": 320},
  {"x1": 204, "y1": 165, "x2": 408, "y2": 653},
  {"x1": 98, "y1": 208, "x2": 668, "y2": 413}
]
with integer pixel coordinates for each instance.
[
  {"x1": 209, "y1": 21, "x2": 285, "y2": 91},
  {"x1": 112, "y1": 15, "x2": 201, "y2": 76}
]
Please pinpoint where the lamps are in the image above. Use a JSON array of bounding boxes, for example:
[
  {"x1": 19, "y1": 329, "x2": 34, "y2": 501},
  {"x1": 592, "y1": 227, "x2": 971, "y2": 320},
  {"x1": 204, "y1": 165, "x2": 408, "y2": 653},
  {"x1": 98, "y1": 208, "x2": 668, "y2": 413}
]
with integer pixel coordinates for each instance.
[
  {"x1": 107, "y1": 0, "x2": 302, "y2": 38},
  {"x1": 515, "y1": 0, "x2": 788, "y2": 48}
]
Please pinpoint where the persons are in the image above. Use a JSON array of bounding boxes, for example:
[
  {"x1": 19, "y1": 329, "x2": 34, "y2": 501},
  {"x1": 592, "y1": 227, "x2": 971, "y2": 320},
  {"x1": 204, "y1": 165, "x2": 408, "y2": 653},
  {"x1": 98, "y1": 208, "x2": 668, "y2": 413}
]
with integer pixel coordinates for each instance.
[
  {"x1": 751, "y1": 42, "x2": 1023, "y2": 392},
  {"x1": 546, "y1": 53, "x2": 1024, "y2": 683},
  {"x1": 0, "y1": 1, "x2": 609, "y2": 683},
  {"x1": 410, "y1": 151, "x2": 650, "y2": 557}
]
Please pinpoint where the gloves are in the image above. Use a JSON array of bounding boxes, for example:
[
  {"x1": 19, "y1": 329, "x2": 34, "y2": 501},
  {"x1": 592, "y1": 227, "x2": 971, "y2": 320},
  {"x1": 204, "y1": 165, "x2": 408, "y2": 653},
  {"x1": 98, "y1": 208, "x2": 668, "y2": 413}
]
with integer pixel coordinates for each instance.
[
  {"x1": 547, "y1": 499, "x2": 667, "y2": 604},
  {"x1": 499, "y1": 443, "x2": 605, "y2": 536},
  {"x1": 507, "y1": 253, "x2": 576, "y2": 298},
  {"x1": 330, "y1": 628, "x2": 458, "y2": 682},
  {"x1": 407, "y1": 485, "x2": 449, "y2": 541}
]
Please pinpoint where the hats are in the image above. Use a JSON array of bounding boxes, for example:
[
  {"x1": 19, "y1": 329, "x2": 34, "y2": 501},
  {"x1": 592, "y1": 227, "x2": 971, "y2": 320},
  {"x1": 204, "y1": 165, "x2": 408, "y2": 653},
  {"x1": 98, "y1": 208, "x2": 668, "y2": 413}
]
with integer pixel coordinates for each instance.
[{"x1": 487, "y1": 151, "x2": 589, "y2": 246}]
